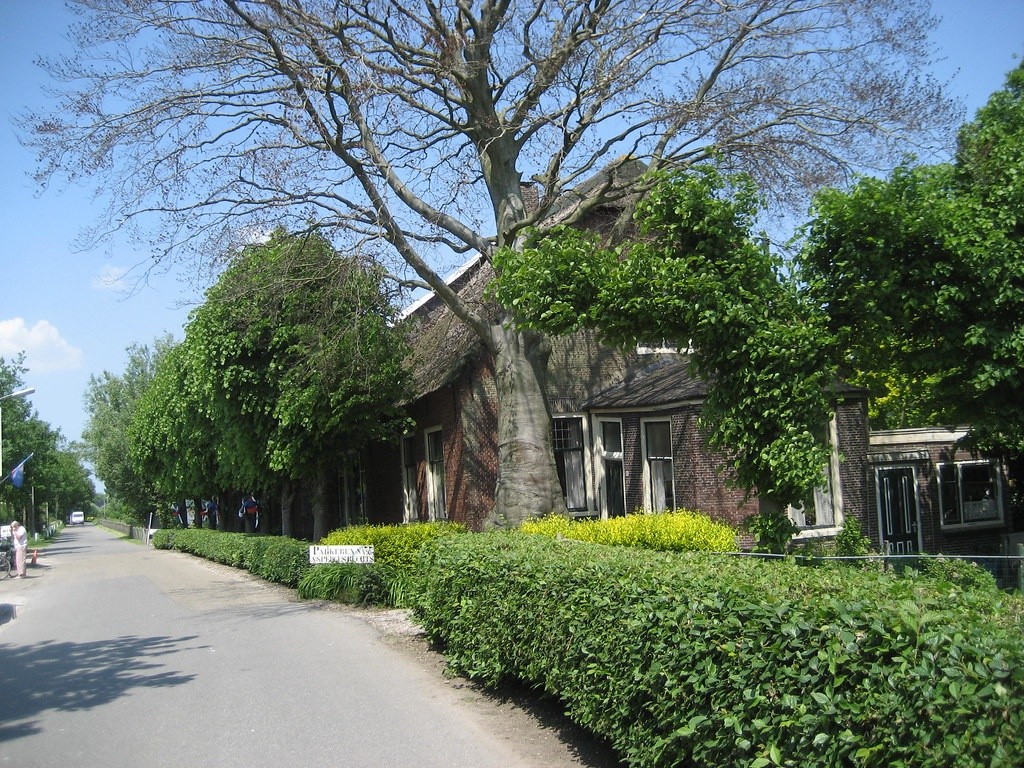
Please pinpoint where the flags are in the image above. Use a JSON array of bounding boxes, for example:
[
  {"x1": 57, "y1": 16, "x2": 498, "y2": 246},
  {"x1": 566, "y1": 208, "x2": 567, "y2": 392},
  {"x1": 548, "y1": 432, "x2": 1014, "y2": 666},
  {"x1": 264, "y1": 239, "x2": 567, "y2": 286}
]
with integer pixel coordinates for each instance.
[{"x1": 10, "y1": 454, "x2": 32, "y2": 488}]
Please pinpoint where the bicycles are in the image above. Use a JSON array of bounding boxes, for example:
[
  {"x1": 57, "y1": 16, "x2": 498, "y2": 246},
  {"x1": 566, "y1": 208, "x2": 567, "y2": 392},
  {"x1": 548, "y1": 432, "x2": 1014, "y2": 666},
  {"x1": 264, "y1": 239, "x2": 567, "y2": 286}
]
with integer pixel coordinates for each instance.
[{"x1": 0, "y1": 539, "x2": 19, "y2": 581}]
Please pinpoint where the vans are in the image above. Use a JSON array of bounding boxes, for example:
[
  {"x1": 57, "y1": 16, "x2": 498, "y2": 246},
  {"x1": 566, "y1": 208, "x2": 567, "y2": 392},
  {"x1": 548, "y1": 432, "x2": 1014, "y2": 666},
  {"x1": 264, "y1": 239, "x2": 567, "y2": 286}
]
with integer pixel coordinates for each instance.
[{"x1": 71, "y1": 511, "x2": 85, "y2": 525}]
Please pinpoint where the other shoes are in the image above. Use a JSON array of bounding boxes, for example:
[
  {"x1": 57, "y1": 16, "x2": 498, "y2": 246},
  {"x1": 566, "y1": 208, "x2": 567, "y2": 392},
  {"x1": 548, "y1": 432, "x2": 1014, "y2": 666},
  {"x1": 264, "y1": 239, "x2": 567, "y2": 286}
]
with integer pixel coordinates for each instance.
[{"x1": 14, "y1": 573, "x2": 27, "y2": 579}]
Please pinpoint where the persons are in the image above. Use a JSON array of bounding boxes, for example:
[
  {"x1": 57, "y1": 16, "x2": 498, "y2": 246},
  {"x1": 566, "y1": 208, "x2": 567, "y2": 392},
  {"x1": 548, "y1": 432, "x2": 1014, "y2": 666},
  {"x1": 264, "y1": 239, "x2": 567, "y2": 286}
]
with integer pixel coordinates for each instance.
[{"x1": 11, "y1": 521, "x2": 28, "y2": 579}]
